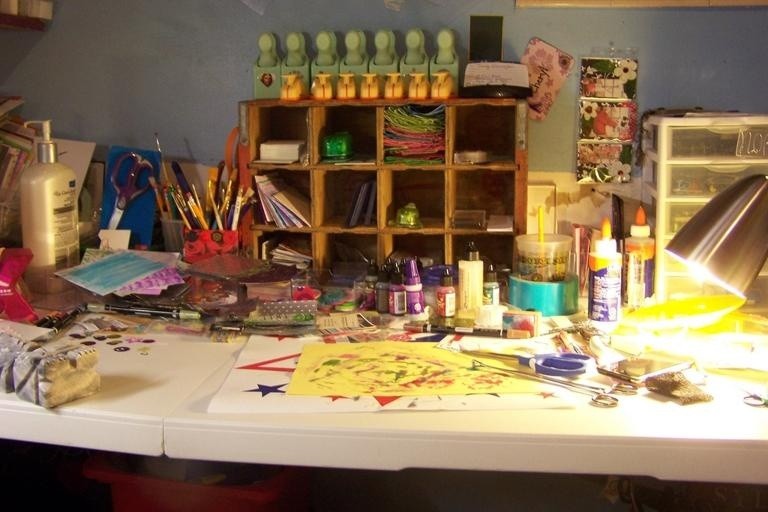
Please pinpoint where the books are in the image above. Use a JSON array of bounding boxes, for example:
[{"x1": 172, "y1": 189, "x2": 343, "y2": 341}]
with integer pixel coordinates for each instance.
[
  {"x1": 344, "y1": 179, "x2": 376, "y2": 227},
  {"x1": 269, "y1": 239, "x2": 315, "y2": 271}
]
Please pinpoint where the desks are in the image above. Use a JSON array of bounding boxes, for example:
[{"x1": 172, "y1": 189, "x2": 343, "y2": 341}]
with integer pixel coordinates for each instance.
[
  {"x1": 163, "y1": 282, "x2": 767, "y2": 492},
  {"x1": 1, "y1": 330, "x2": 246, "y2": 458}
]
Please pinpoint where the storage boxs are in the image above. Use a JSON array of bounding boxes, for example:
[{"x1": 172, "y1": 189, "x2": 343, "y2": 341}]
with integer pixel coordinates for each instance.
[{"x1": 78, "y1": 446, "x2": 304, "y2": 510}]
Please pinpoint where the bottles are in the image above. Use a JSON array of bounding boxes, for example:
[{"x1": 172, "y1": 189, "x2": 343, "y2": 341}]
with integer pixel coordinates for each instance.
[
  {"x1": 585, "y1": 214, "x2": 623, "y2": 329},
  {"x1": 621, "y1": 205, "x2": 656, "y2": 307},
  {"x1": 362, "y1": 242, "x2": 501, "y2": 318}
]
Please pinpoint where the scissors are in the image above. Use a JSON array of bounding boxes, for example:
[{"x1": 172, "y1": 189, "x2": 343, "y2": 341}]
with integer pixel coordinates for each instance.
[
  {"x1": 460, "y1": 348, "x2": 595, "y2": 376},
  {"x1": 473, "y1": 359, "x2": 640, "y2": 408},
  {"x1": 225, "y1": 128, "x2": 239, "y2": 202},
  {"x1": 107, "y1": 151, "x2": 154, "y2": 231}
]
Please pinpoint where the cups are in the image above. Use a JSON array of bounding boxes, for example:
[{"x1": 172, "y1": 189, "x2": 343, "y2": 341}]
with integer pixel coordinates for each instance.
[
  {"x1": 515, "y1": 232, "x2": 574, "y2": 281},
  {"x1": 183, "y1": 226, "x2": 240, "y2": 306},
  {"x1": 159, "y1": 217, "x2": 186, "y2": 252}
]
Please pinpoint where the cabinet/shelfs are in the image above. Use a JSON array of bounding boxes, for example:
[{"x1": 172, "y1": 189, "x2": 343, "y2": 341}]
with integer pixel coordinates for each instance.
[
  {"x1": 635, "y1": 108, "x2": 768, "y2": 307},
  {"x1": 231, "y1": 97, "x2": 531, "y2": 285}
]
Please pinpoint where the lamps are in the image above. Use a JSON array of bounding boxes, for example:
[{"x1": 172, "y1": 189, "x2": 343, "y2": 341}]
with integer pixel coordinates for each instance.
[{"x1": 665, "y1": 171, "x2": 768, "y2": 301}]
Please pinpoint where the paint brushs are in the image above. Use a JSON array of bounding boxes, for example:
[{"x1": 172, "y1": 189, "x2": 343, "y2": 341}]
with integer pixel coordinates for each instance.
[{"x1": 149, "y1": 161, "x2": 257, "y2": 230}]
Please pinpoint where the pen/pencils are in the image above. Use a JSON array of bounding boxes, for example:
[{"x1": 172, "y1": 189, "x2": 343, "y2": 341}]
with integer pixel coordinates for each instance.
[
  {"x1": 86, "y1": 300, "x2": 201, "y2": 319},
  {"x1": 33, "y1": 303, "x2": 88, "y2": 335},
  {"x1": 404, "y1": 323, "x2": 531, "y2": 338},
  {"x1": 538, "y1": 207, "x2": 545, "y2": 281}
]
